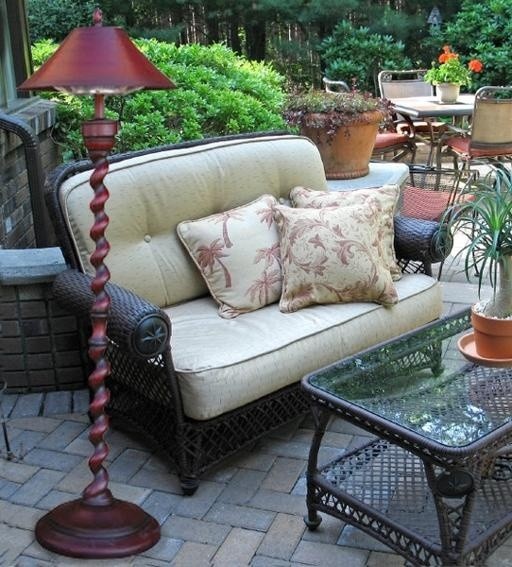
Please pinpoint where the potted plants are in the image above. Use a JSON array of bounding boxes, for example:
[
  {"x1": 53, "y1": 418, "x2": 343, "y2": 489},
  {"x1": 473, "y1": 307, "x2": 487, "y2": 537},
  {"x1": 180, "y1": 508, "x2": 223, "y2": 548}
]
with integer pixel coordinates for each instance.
[
  {"x1": 282, "y1": 93, "x2": 397, "y2": 179},
  {"x1": 438, "y1": 157, "x2": 512, "y2": 359}
]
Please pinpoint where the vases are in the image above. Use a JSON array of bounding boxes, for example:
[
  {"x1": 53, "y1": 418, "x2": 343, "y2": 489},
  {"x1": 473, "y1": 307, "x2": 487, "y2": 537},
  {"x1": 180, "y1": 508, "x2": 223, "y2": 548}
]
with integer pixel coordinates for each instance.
[{"x1": 436, "y1": 84, "x2": 459, "y2": 105}]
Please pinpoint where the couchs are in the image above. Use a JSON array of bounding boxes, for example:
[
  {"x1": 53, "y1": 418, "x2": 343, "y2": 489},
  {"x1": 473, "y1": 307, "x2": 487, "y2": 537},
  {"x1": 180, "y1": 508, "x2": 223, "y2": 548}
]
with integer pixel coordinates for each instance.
[{"x1": 43, "y1": 131, "x2": 453, "y2": 496}]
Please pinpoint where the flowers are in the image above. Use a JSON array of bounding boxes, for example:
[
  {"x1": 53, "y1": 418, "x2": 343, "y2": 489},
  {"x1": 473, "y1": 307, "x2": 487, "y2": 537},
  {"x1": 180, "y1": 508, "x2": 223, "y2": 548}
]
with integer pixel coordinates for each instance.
[{"x1": 424, "y1": 45, "x2": 482, "y2": 89}]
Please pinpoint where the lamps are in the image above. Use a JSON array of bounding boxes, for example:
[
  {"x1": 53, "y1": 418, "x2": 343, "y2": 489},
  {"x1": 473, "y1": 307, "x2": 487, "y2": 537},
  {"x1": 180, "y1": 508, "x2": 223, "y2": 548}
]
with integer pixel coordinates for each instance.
[{"x1": 16, "y1": 27, "x2": 177, "y2": 559}]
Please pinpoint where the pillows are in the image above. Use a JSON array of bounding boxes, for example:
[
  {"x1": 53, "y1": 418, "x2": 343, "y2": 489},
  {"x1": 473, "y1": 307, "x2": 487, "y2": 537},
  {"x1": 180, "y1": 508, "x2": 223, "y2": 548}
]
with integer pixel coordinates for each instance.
[
  {"x1": 176, "y1": 194, "x2": 281, "y2": 320},
  {"x1": 271, "y1": 195, "x2": 398, "y2": 314},
  {"x1": 289, "y1": 183, "x2": 403, "y2": 282}
]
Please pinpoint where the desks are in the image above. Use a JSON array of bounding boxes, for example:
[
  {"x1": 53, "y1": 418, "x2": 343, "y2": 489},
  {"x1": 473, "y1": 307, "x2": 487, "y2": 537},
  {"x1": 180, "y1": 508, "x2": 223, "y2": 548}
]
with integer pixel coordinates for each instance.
[{"x1": 390, "y1": 96, "x2": 487, "y2": 188}]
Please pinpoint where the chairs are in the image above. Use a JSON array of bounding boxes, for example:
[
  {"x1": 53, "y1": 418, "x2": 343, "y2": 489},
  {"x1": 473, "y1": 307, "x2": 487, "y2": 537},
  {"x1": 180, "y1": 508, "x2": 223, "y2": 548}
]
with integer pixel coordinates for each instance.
[
  {"x1": 420, "y1": 86, "x2": 512, "y2": 191},
  {"x1": 322, "y1": 77, "x2": 417, "y2": 187},
  {"x1": 378, "y1": 70, "x2": 461, "y2": 188}
]
